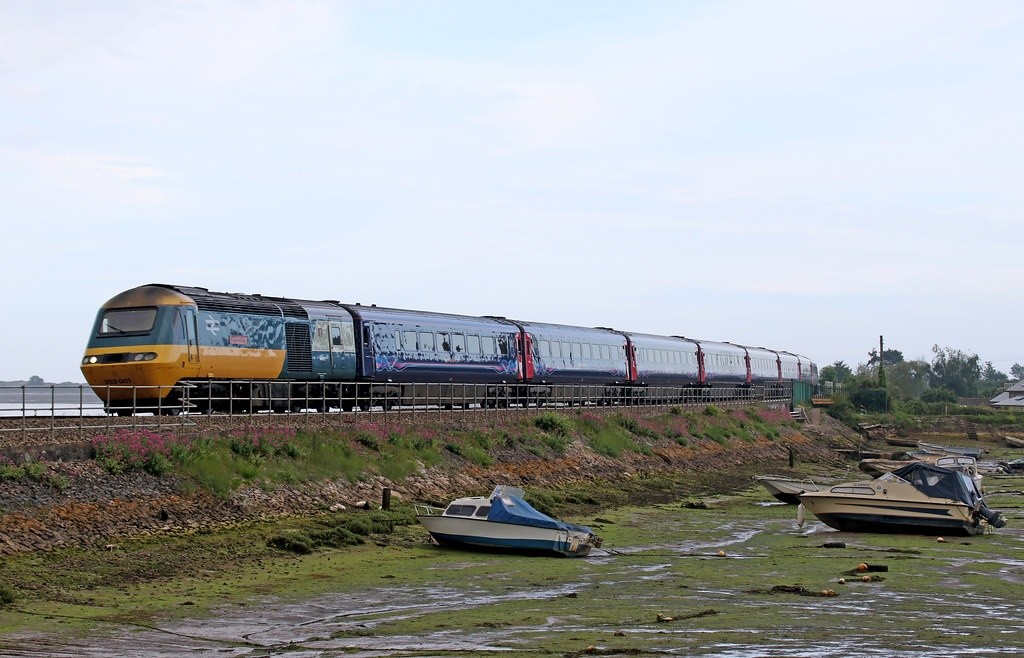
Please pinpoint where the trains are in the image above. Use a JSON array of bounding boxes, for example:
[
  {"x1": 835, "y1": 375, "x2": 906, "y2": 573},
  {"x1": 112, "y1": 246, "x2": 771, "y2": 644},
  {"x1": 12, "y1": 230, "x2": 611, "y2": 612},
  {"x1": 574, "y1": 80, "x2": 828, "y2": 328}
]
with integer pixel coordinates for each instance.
[{"x1": 80, "y1": 283, "x2": 820, "y2": 415}]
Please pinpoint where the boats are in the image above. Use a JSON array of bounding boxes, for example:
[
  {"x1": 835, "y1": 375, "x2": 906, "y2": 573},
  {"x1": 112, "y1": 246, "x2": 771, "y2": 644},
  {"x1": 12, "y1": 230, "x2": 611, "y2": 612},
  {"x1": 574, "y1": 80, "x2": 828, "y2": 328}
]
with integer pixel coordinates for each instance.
[
  {"x1": 796, "y1": 462, "x2": 1007, "y2": 537},
  {"x1": 834, "y1": 442, "x2": 1024, "y2": 491},
  {"x1": 1004, "y1": 435, "x2": 1024, "y2": 450},
  {"x1": 886, "y1": 436, "x2": 923, "y2": 448},
  {"x1": 752, "y1": 473, "x2": 849, "y2": 507},
  {"x1": 414, "y1": 483, "x2": 604, "y2": 560}
]
